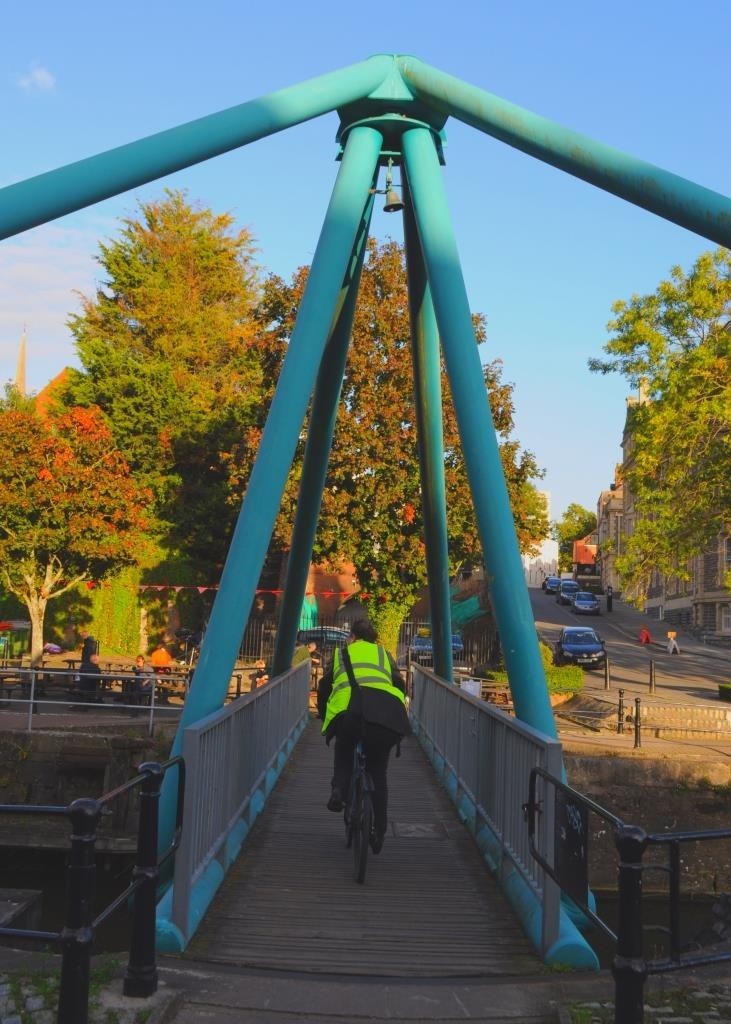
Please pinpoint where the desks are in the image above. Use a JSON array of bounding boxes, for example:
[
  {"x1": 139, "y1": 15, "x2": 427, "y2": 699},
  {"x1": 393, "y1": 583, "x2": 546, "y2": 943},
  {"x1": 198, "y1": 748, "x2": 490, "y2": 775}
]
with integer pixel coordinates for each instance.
[{"x1": 482, "y1": 687, "x2": 511, "y2": 715}]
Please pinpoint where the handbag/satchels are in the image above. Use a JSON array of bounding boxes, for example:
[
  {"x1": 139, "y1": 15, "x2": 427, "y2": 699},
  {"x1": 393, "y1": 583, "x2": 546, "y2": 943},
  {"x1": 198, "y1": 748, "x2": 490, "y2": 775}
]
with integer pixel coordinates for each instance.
[{"x1": 342, "y1": 686, "x2": 408, "y2": 745}]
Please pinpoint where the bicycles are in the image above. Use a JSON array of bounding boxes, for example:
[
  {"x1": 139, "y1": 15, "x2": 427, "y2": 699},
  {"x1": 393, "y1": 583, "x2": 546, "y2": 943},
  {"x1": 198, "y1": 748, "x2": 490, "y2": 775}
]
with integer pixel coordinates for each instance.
[{"x1": 330, "y1": 738, "x2": 381, "y2": 884}]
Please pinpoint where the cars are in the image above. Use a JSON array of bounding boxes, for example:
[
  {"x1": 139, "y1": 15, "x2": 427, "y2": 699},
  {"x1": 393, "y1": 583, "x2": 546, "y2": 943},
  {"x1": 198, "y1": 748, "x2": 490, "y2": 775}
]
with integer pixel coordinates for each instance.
[
  {"x1": 554, "y1": 626, "x2": 608, "y2": 670},
  {"x1": 570, "y1": 591, "x2": 601, "y2": 616},
  {"x1": 451, "y1": 634, "x2": 464, "y2": 660},
  {"x1": 556, "y1": 581, "x2": 580, "y2": 605},
  {"x1": 297, "y1": 624, "x2": 350, "y2": 664},
  {"x1": 405, "y1": 635, "x2": 434, "y2": 666},
  {"x1": 541, "y1": 576, "x2": 557, "y2": 590},
  {"x1": 546, "y1": 578, "x2": 561, "y2": 594}
]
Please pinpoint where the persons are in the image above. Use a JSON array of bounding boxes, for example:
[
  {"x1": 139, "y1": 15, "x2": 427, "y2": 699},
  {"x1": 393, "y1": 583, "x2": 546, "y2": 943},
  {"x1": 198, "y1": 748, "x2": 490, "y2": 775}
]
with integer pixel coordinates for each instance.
[
  {"x1": 80, "y1": 631, "x2": 199, "y2": 706},
  {"x1": 318, "y1": 620, "x2": 409, "y2": 856},
  {"x1": 293, "y1": 640, "x2": 320, "y2": 669}
]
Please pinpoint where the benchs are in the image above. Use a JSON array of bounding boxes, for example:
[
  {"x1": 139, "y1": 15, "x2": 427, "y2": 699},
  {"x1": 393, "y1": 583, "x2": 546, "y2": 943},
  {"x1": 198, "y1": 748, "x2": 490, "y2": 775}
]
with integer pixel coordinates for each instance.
[
  {"x1": 65, "y1": 688, "x2": 157, "y2": 713},
  {"x1": 0, "y1": 657, "x2": 195, "y2": 678},
  {"x1": 493, "y1": 703, "x2": 514, "y2": 711}
]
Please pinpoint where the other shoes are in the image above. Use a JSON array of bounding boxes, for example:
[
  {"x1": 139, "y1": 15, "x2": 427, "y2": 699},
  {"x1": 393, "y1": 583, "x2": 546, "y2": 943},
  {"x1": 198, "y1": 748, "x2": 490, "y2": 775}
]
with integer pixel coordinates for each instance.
[
  {"x1": 372, "y1": 832, "x2": 384, "y2": 853},
  {"x1": 327, "y1": 787, "x2": 343, "y2": 813}
]
